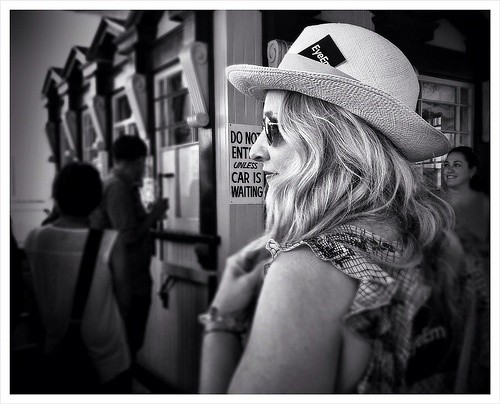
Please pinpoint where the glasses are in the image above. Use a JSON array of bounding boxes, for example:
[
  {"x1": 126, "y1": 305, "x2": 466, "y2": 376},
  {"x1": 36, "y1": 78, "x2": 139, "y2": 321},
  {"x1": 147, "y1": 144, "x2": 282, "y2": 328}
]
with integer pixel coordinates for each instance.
[{"x1": 260, "y1": 117, "x2": 279, "y2": 146}]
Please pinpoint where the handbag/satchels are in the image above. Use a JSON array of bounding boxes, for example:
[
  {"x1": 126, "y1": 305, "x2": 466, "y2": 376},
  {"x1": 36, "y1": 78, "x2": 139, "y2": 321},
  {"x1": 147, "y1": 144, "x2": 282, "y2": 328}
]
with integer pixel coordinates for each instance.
[{"x1": 48, "y1": 331, "x2": 100, "y2": 394}]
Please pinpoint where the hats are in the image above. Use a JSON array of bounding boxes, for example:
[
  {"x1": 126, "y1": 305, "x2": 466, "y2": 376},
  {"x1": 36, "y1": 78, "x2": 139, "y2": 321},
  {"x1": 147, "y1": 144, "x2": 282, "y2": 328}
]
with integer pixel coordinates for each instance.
[{"x1": 225, "y1": 22, "x2": 452, "y2": 161}]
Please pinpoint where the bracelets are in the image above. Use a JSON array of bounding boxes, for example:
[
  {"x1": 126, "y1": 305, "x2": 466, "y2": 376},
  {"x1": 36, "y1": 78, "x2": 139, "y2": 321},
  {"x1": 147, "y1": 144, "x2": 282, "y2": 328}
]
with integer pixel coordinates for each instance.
[
  {"x1": 199, "y1": 307, "x2": 248, "y2": 329},
  {"x1": 202, "y1": 327, "x2": 239, "y2": 335}
]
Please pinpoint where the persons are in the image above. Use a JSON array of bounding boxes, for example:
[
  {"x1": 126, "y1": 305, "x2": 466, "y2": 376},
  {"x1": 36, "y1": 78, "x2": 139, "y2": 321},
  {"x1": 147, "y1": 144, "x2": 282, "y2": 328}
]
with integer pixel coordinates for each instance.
[
  {"x1": 23, "y1": 134, "x2": 170, "y2": 393},
  {"x1": 199, "y1": 22, "x2": 489, "y2": 394}
]
